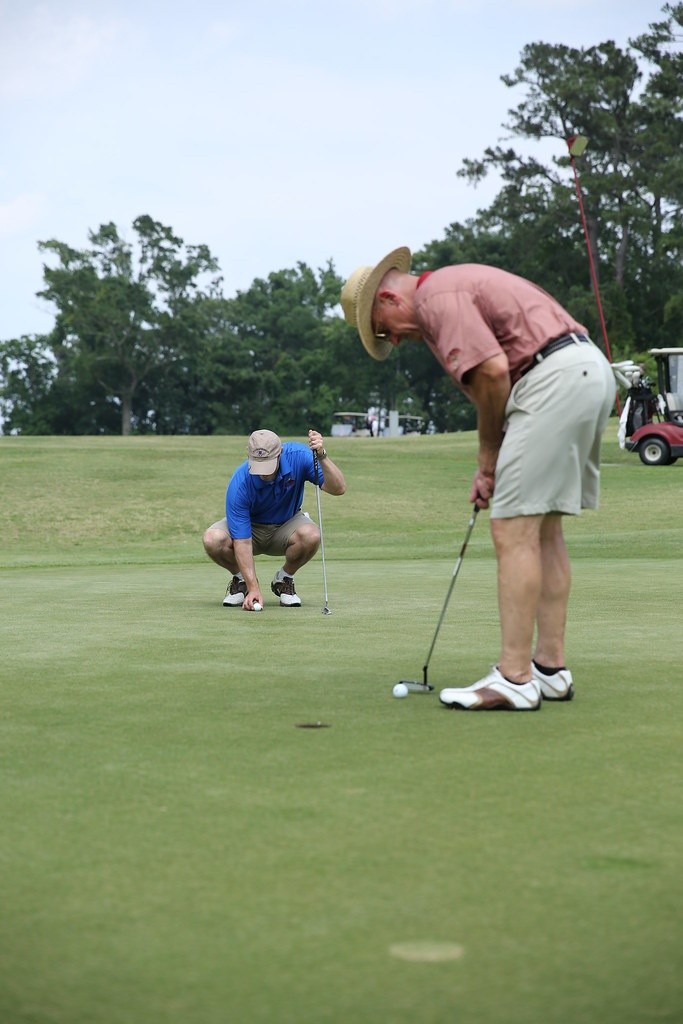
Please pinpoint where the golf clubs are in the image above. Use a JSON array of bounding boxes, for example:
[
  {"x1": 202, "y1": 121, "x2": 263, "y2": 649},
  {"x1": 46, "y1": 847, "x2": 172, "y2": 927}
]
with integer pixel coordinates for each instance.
[
  {"x1": 313, "y1": 449, "x2": 332, "y2": 615},
  {"x1": 398, "y1": 490, "x2": 482, "y2": 692}
]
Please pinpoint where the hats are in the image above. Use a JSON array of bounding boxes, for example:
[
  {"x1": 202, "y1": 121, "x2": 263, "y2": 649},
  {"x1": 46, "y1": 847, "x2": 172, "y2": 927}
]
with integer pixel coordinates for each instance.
[
  {"x1": 340, "y1": 246, "x2": 411, "y2": 361},
  {"x1": 247, "y1": 430, "x2": 282, "y2": 476}
]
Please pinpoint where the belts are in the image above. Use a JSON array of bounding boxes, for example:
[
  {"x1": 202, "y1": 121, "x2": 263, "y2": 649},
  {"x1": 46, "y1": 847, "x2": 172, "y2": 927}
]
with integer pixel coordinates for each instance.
[{"x1": 520, "y1": 332, "x2": 588, "y2": 377}]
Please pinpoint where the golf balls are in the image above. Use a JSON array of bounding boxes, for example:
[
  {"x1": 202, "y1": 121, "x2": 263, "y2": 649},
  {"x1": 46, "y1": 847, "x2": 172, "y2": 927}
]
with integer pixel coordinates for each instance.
[
  {"x1": 391, "y1": 683, "x2": 408, "y2": 698},
  {"x1": 254, "y1": 602, "x2": 262, "y2": 611}
]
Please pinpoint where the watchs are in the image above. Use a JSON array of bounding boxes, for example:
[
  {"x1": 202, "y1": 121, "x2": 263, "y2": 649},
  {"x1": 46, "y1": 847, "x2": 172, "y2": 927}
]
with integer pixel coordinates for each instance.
[{"x1": 316, "y1": 450, "x2": 327, "y2": 461}]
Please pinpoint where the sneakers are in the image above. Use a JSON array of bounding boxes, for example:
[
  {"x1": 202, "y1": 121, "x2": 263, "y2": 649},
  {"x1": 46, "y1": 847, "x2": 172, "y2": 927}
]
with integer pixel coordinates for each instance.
[
  {"x1": 439, "y1": 661, "x2": 575, "y2": 711},
  {"x1": 270, "y1": 571, "x2": 302, "y2": 607},
  {"x1": 222, "y1": 576, "x2": 258, "y2": 607}
]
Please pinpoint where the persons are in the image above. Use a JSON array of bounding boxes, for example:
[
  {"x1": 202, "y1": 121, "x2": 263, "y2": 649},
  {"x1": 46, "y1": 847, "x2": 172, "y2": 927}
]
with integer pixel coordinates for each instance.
[
  {"x1": 364, "y1": 401, "x2": 387, "y2": 437},
  {"x1": 338, "y1": 243, "x2": 617, "y2": 713},
  {"x1": 202, "y1": 429, "x2": 347, "y2": 608}
]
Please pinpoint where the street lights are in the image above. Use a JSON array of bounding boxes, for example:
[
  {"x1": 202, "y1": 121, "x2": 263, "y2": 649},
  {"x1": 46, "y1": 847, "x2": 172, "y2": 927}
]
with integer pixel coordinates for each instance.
[{"x1": 566, "y1": 135, "x2": 622, "y2": 416}]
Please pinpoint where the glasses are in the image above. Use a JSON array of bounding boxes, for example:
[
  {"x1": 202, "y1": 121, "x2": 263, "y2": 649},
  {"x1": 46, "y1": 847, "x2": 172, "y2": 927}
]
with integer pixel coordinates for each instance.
[{"x1": 372, "y1": 293, "x2": 391, "y2": 342}]
